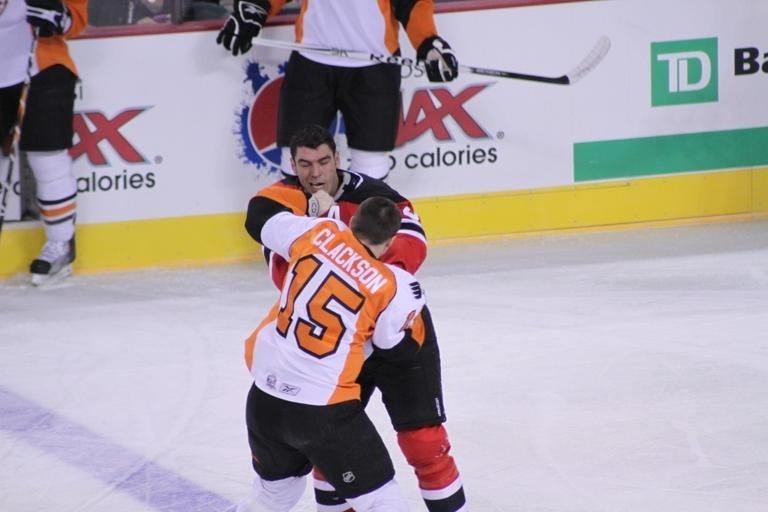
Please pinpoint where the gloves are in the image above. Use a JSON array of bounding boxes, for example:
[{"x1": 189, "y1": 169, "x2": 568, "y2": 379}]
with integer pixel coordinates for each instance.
[
  {"x1": 416, "y1": 34, "x2": 458, "y2": 82},
  {"x1": 216, "y1": 1, "x2": 271, "y2": 56},
  {"x1": 25, "y1": 0, "x2": 70, "y2": 38}
]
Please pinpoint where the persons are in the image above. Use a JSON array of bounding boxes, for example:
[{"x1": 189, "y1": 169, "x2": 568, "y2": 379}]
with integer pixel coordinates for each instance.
[
  {"x1": 0, "y1": 0, "x2": 90, "y2": 292},
  {"x1": 214, "y1": 0, "x2": 459, "y2": 185},
  {"x1": 236, "y1": 180, "x2": 427, "y2": 512},
  {"x1": 259, "y1": 122, "x2": 478, "y2": 512}
]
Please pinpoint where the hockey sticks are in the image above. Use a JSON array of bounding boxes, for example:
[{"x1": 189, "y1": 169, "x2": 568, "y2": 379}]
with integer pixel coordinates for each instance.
[{"x1": 251, "y1": 35, "x2": 610, "y2": 86}]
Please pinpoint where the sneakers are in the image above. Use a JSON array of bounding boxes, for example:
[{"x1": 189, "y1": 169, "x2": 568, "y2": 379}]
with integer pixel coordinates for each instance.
[{"x1": 30, "y1": 237, "x2": 76, "y2": 274}]
